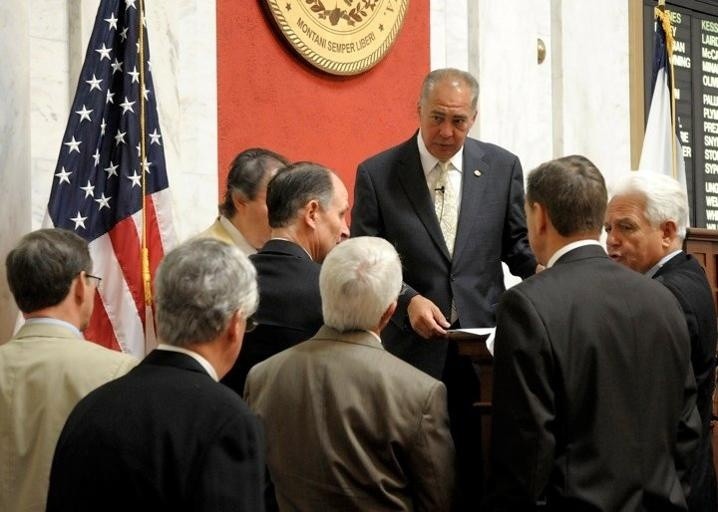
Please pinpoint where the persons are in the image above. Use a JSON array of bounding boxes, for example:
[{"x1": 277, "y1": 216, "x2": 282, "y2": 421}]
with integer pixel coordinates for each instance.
[
  {"x1": 350, "y1": 67, "x2": 542, "y2": 510},
  {"x1": 486, "y1": 150, "x2": 702, "y2": 511},
  {"x1": 603, "y1": 168, "x2": 718, "y2": 442},
  {"x1": 42, "y1": 234, "x2": 274, "y2": 510},
  {"x1": 212, "y1": 160, "x2": 351, "y2": 397},
  {"x1": 243, "y1": 234, "x2": 458, "y2": 509},
  {"x1": 198, "y1": 146, "x2": 290, "y2": 254},
  {"x1": 0, "y1": 228, "x2": 138, "y2": 510}
]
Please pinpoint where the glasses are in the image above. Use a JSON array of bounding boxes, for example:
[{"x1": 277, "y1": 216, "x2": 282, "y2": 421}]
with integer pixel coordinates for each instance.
[{"x1": 75, "y1": 271, "x2": 101, "y2": 288}]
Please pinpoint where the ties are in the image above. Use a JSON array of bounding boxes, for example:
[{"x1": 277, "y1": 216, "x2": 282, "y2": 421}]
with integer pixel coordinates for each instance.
[{"x1": 432, "y1": 162, "x2": 456, "y2": 255}]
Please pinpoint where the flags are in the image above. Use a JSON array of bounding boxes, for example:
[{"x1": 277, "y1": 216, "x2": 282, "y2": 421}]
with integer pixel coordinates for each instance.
[
  {"x1": 39, "y1": 0, "x2": 172, "y2": 358},
  {"x1": 632, "y1": 3, "x2": 690, "y2": 240}
]
